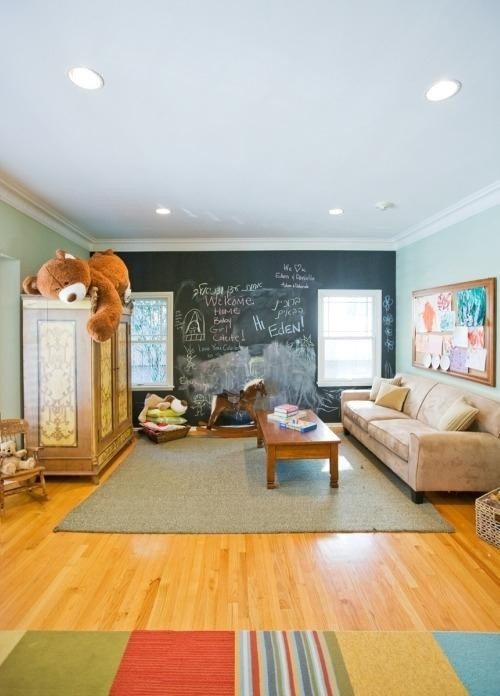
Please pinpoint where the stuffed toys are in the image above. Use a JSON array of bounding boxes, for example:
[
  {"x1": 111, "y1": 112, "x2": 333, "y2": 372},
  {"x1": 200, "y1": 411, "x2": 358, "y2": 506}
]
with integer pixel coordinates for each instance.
[
  {"x1": 22, "y1": 246, "x2": 134, "y2": 343},
  {"x1": 0, "y1": 440, "x2": 38, "y2": 476},
  {"x1": 206, "y1": 376, "x2": 268, "y2": 430},
  {"x1": 138, "y1": 392, "x2": 189, "y2": 423}
]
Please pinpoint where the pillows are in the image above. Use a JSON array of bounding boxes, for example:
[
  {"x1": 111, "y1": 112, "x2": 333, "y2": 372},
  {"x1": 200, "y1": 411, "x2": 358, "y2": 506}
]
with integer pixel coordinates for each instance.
[
  {"x1": 151, "y1": 416, "x2": 189, "y2": 425},
  {"x1": 139, "y1": 422, "x2": 186, "y2": 432},
  {"x1": 375, "y1": 381, "x2": 411, "y2": 411},
  {"x1": 435, "y1": 395, "x2": 481, "y2": 431},
  {"x1": 146, "y1": 407, "x2": 176, "y2": 417},
  {"x1": 369, "y1": 375, "x2": 402, "y2": 401}
]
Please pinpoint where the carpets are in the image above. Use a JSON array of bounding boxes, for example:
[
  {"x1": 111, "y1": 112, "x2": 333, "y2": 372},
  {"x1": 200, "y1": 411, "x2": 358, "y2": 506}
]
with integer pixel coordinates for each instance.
[
  {"x1": 52, "y1": 432, "x2": 458, "y2": 533},
  {"x1": 0, "y1": 628, "x2": 500, "y2": 695}
]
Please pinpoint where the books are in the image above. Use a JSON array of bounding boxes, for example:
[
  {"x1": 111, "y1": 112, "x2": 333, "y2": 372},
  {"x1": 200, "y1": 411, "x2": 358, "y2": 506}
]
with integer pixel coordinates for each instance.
[{"x1": 266, "y1": 401, "x2": 317, "y2": 434}]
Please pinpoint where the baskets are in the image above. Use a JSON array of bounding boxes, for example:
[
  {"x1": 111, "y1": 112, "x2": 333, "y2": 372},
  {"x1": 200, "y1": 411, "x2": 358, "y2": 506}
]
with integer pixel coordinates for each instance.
[
  {"x1": 475, "y1": 487, "x2": 500, "y2": 549},
  {"x1": 142, "y1": 424, "x2": 191, "y2": 444}
]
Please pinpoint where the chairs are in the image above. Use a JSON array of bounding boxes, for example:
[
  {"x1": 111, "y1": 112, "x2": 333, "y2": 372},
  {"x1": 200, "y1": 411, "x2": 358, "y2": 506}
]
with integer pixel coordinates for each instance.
[{"x1": 0, "y1": 418, "x2": 51, "y2": 510}]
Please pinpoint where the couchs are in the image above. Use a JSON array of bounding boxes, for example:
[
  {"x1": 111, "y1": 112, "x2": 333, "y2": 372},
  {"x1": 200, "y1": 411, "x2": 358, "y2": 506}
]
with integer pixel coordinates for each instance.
[{"x1": 337, "y1": 371, "x2": 500, "y2": 504}]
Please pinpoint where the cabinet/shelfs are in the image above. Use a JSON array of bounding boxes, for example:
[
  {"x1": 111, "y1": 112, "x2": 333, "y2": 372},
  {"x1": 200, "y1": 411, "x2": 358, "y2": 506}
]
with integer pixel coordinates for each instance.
[{"x1": 21, "y1": 293, "x2": 134, "y2": 484}]
